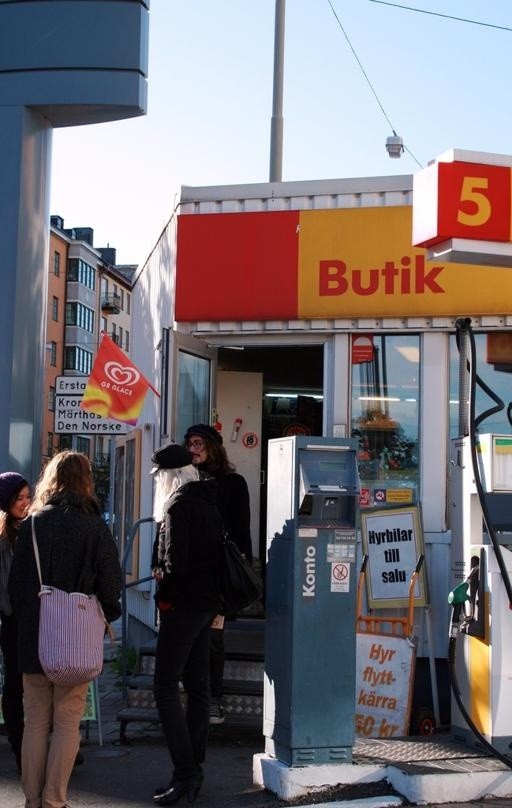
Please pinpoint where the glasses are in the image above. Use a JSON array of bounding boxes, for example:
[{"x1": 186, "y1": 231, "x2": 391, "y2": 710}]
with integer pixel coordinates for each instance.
[{"x1": 186, "y1": 441, "x2": 203, "y2": 449}]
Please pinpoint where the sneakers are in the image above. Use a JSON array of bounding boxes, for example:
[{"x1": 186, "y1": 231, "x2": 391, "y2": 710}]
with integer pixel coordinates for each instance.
[{"x1": 209, "y1": 702, "x2": 226, "y2": 725}]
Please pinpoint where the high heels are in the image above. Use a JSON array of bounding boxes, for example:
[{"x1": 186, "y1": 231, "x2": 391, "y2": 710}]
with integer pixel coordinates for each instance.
[{"x1": 153, "y1": 773, "x2": 202, "y2": 807}]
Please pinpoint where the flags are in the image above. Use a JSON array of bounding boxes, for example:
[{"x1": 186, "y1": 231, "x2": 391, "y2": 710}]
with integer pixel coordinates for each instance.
[{"x1": 79, "y1": 335, "x2": 149, "y2": 426}]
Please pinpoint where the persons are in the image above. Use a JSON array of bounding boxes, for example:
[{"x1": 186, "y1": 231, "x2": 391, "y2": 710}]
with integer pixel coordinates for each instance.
[
  {"x1": 0, "y1": 469, "x2": 33, "y2": 769},
  {"x1": 147, "y1": 441, "x2": 228, "y2": 805},
  {"x1": 184, "y1": 423, "x2": 255, "y2": 725},
  {"x1": 7, "y1": 450, "x2": 126, "y2": 808}
]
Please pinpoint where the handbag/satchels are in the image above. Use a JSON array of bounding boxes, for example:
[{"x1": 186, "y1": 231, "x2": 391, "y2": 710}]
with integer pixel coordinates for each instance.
[
  {"x1": 219, "y1": 532, "x2": 263, "y2": 618},
  {"x1": 39, "y1": 584, "x2": 108, "y2": 689}
]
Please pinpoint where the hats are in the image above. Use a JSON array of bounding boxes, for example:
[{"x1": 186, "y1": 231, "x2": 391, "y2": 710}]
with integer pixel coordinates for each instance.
[
  {"x1": 0, "y1": 472, "x2": 25, "y2": 507},
  {"x1": 148, "y1": 444, "x2": 193, "y2": 475},
  {"x1": 184, "y1": 424, "x2": 223, "y2": 444}
]
